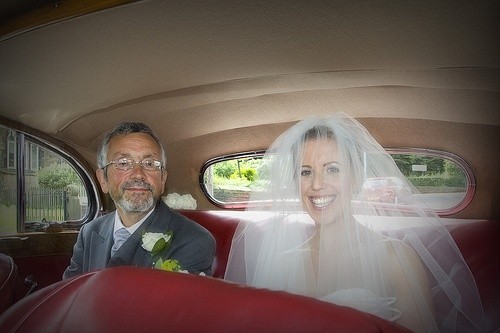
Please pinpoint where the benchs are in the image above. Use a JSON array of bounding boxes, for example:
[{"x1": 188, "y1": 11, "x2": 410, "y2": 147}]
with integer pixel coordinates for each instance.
[{"x1": 174, "y1": 209, "x2": 500, "y2": 333}]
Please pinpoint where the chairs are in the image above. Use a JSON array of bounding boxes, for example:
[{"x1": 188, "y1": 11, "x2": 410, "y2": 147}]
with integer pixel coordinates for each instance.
[{"x1": 0, "y1": 266, "x2": 414, "y2": 333}]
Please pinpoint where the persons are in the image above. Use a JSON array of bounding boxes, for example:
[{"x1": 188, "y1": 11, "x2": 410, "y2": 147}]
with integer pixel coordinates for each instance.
[
  {"x1": 62, "y1": 123, "x2": 217, "y2": 279},
  {"x1": 224, "y1": 111, "x2": 487, "y2": 333}
]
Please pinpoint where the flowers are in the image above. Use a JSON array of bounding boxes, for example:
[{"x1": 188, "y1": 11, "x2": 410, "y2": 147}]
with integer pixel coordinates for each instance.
[
  {"x1": 140, "y1": 229, "x2": 182, "y2": 273},
  {"x1": 161, "y1": 193, "x2": 198, "y2": 211}
]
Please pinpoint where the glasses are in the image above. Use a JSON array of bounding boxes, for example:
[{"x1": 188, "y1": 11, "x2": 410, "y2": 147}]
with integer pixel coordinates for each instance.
[{"x1": 100, "y1": 155, "x2": 163, "y2": 173}]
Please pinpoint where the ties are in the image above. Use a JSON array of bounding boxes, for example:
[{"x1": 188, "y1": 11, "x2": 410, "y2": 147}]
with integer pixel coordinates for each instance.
[{"x1": 110, "y1": 228, "x2": 133, "y2": 258}]
